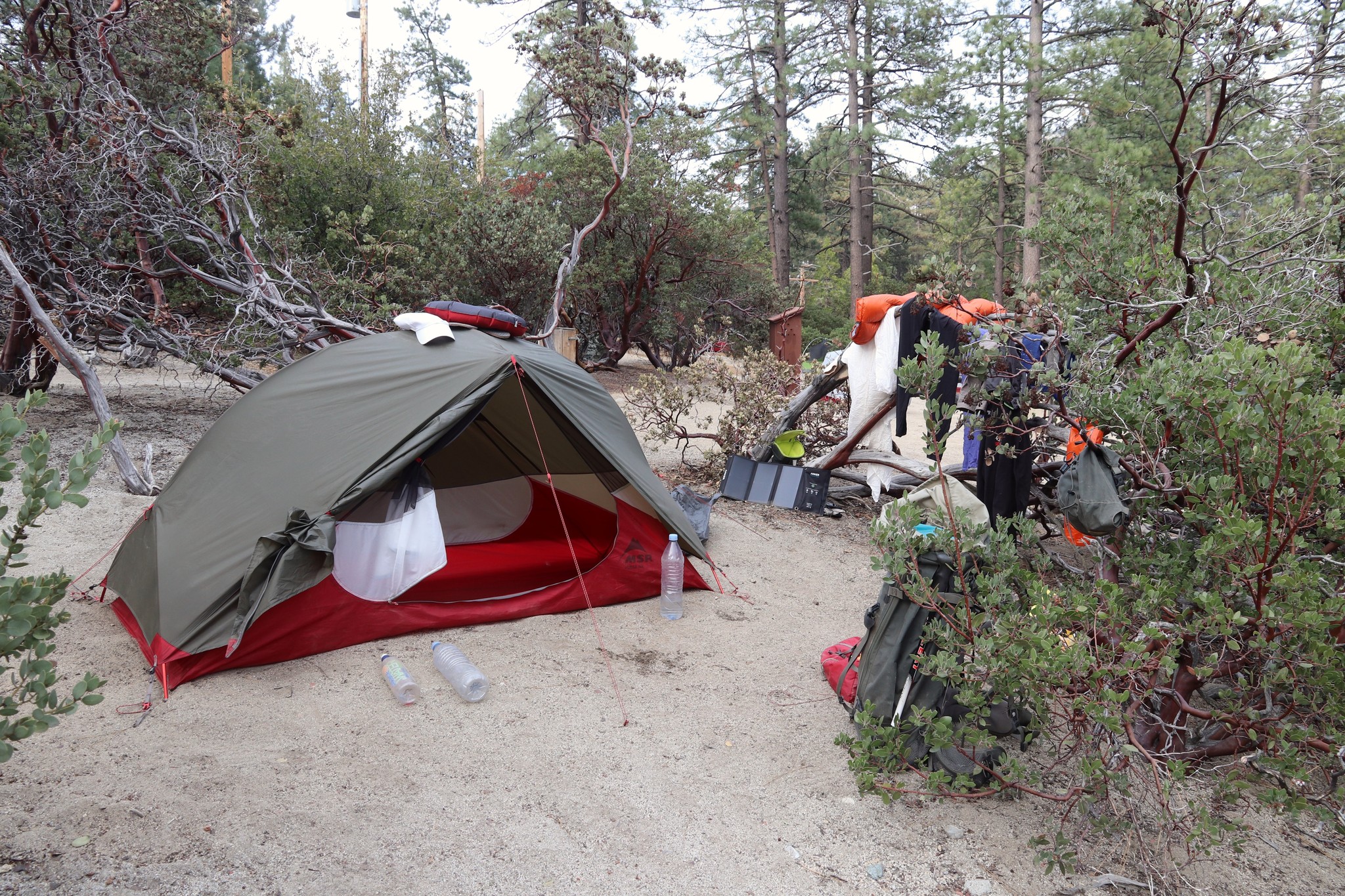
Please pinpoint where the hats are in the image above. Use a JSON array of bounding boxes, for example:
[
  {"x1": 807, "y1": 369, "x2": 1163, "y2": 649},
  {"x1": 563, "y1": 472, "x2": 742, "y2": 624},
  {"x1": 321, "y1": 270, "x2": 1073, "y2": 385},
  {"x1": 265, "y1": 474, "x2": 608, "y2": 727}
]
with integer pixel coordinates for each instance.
[{"x1": 393, "y1": 312, "x2": 455, "y2": 344}]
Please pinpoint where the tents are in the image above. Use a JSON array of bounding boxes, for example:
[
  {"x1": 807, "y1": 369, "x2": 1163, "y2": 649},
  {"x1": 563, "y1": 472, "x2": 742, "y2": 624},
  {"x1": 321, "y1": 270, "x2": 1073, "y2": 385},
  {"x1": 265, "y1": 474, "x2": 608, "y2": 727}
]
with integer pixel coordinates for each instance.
[{"x1": 99, "y1": 322, "x2": 723, "y2": 690}]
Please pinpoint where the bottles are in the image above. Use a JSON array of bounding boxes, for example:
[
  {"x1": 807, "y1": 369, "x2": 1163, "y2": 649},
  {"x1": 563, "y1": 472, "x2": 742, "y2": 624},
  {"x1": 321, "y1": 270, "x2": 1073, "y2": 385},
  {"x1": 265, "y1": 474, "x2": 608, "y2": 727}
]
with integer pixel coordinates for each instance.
[
  {"x1": 380, "y1": 654, "x2": 420, "y2": 705},
  {"x1": 430, "y1": 641, "x2": 488, "y2": 702},
  {"x1": 660, "y1": 534, "x2": 684, "y2": 620}
]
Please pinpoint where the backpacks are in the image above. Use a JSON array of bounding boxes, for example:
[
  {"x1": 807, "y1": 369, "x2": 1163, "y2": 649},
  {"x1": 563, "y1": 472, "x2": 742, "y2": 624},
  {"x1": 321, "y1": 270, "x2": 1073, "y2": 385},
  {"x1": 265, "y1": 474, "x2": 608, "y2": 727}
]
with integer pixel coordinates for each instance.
[{"x1": 836, "y1": 541, "x2": 995, "y2": 767}]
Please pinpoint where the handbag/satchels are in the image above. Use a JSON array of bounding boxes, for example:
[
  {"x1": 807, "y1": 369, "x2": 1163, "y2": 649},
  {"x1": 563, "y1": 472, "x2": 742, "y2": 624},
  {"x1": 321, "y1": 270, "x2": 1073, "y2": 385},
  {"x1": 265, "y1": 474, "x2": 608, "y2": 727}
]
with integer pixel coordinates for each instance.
[
  {"x1": 1056, "y1": 443, "x2": 1130, "y2": 537},
  {"x1": 820, "y1": 633, "x2": 863, "y2": 703}
]
